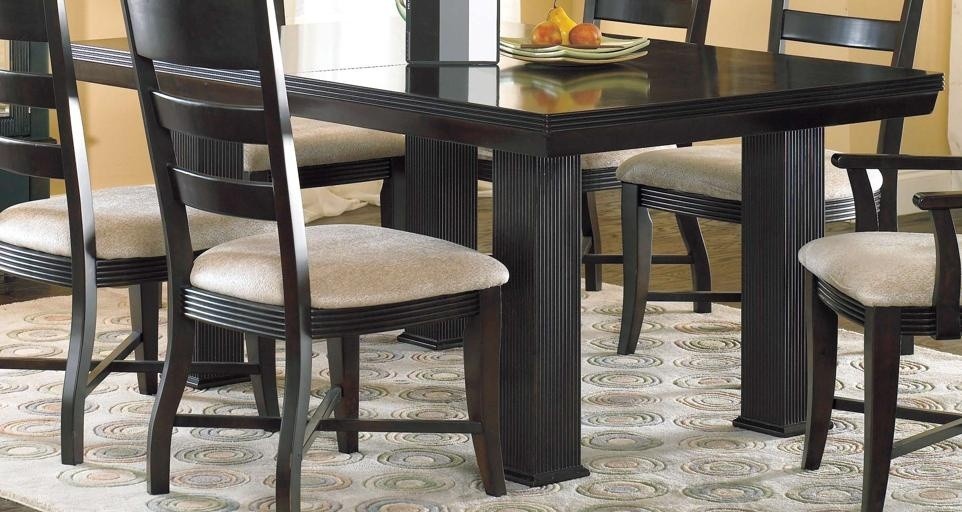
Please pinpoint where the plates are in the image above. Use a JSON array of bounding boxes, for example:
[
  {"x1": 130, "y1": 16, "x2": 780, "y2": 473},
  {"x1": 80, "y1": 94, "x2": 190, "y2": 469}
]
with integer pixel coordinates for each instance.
[{"x1": 498, "y1": 35, "x2": 652, "y2": 68}]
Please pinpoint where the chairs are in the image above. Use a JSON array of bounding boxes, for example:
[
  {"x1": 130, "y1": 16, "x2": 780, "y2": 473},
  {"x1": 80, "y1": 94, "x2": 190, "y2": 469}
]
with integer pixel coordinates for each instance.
[
  {"x1": 796, "y1": 154, "x2": 961, "y2": 512},
  {"x1": 0, "y1": 0, "x2": 285, "y2": 466},
  {"x1": 121, "y1": 1, "x2": 511, "y2": 511},
  {"x1": 614, "y1": 0, "x2": 926, "y2": 354},
  {"x1": 437, "y1": 0, "x2": 711, "y2": 313}
]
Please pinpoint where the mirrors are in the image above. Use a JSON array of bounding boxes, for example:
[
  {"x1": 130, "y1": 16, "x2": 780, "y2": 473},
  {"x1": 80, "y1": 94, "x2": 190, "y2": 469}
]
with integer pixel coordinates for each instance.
[
  {"x1": 403, "y1": 0, "x2": 501, "y2": 67},
  {"x1": 405, "y1": 66, "x2": 501, "y2": 105}
]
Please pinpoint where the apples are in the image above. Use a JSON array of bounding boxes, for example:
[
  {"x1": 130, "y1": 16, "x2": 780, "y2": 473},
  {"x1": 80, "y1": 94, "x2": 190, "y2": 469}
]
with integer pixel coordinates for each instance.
[
  {"x1": 531, "y1": 21, "x2": 563, "y2": 46},
  {"x1": 568, "y1": 22, "x2": 601, "y2": 48}
]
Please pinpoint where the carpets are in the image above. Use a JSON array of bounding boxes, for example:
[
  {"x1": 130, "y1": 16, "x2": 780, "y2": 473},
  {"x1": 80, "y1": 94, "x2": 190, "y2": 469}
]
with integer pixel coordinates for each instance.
[{"x1": 1, "y1": 254, "x2": 960, "y2": 511}]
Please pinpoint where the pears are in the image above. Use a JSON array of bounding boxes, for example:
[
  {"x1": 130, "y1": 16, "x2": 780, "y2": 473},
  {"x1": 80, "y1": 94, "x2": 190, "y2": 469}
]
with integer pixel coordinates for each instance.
[{"x1": 546, "y1": 2, "x2": 576, "y2": 47}]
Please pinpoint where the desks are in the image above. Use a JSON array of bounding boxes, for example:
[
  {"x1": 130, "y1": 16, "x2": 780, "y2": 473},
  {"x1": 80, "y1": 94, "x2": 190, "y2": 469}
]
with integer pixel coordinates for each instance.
[{"x1": 71, "y1": 24, "x2": 944, "y2": 489}]
